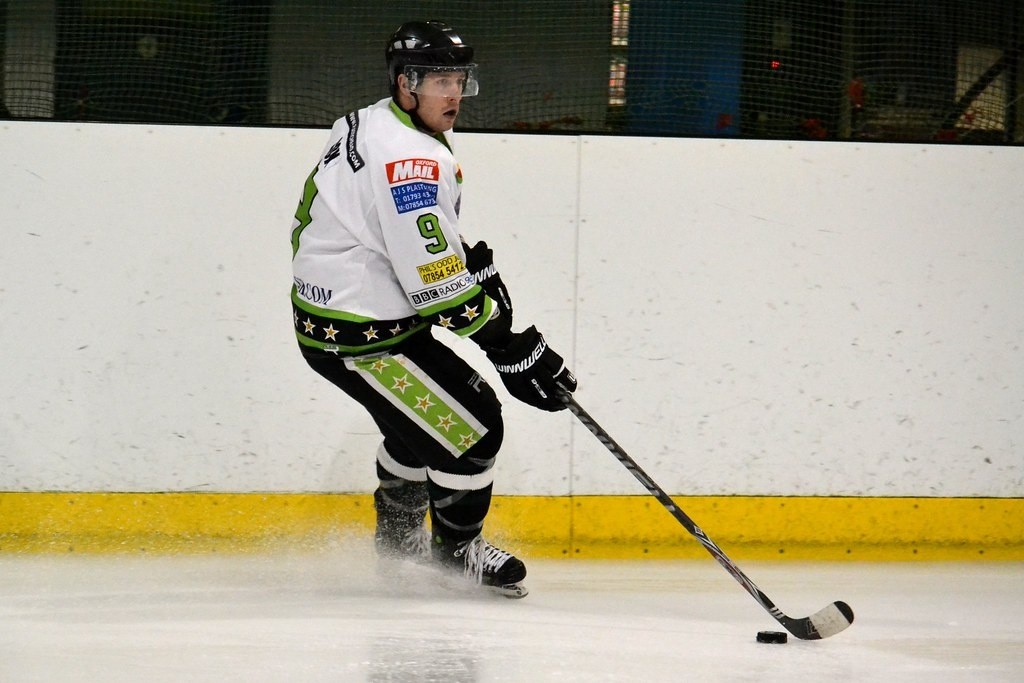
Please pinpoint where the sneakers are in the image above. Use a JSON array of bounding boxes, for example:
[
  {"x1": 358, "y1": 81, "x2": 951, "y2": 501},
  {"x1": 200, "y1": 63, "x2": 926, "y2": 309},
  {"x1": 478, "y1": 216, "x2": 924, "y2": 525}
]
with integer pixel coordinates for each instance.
[
  {"x1": 374, "y1": 489, "x2": 432, "y2": 571},
  {"x1": 428, "y1": 498, "x2": 528, "y2": 598}
]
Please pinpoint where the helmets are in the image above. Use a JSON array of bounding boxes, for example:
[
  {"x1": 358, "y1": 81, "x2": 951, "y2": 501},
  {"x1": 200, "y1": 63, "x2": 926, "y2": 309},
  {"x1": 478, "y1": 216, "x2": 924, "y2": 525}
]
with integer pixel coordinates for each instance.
[{"x1": 385, "y1": 19, "x2": 479, "y2": 97}]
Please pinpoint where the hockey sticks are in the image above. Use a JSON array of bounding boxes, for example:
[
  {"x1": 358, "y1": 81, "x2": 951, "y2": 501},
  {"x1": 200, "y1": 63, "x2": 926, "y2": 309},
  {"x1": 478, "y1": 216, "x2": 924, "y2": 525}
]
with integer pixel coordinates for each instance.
[{"x1": 555, "y1": 380, "x2": 854, "y2": 641}]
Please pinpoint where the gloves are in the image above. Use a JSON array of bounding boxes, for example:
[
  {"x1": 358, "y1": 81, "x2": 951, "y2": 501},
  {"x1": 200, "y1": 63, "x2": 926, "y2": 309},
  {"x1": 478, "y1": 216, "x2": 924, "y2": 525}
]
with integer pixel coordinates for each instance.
[
  {"x1": 486, "y1": 324, "x2": 577, "y2": 412},
  {"x1": 462, "y1": 241, "x2": 512, "y2": 329}
]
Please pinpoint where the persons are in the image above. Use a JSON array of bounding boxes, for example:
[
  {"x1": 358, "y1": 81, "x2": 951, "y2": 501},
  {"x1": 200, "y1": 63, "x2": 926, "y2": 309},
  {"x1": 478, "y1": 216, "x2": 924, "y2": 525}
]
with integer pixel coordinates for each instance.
[{"x1": 290, "y1": 20, "x2": 578, "y2": 599}]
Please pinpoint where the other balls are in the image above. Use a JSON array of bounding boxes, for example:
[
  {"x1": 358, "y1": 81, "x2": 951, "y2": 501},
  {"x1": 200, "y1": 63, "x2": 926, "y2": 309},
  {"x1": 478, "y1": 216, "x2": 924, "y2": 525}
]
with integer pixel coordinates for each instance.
[{"x1": 755, "y1": 631, "x2": 789, "y2": 645}]
